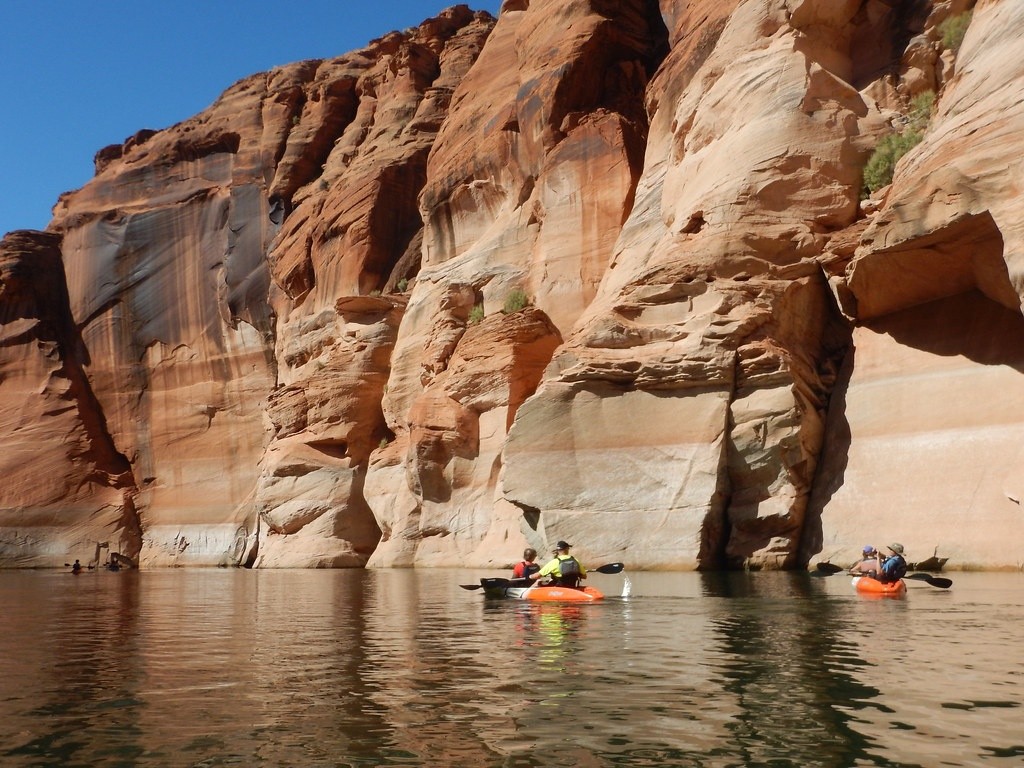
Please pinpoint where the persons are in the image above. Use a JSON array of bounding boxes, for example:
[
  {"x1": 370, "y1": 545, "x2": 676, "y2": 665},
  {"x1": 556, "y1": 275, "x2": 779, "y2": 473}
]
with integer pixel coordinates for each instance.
[
  {"x1": 526, "y1": 540, "x2": 588, "y2": 588},
  {"x1": 512, "y1": 548, "x2": 540, "y2": 588},
  {"x1": 851, "y1": 545, "x2": 877, "y2": 572},
  {"x1": 876, "y1": 543, "x2": 907, "y2": 581}
]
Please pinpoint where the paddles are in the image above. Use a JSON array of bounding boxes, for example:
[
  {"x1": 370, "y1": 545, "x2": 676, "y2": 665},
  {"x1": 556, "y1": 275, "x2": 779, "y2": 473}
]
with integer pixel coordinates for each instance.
[
  {"x1": 64, "y1": 563, "x2": 94, "y2": 570},
  {"x1": 458, "y1": 563, "x2": 624, "y2": 591},
  {"x1": 808, "y1": 562, "x2": 953, "y2": 588}
]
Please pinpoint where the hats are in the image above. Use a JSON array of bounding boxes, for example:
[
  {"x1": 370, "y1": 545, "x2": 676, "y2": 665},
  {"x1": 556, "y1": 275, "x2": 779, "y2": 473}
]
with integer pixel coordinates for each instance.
[
  {"x1": 556, "y1": 541, "x2": 572, "y2": 549},
  {"x1": 886, "y1": 543, "x2": 907, "y2": 556},
  {"x1": 864, "y1": 545, "x2": 874, "y2": 555}
]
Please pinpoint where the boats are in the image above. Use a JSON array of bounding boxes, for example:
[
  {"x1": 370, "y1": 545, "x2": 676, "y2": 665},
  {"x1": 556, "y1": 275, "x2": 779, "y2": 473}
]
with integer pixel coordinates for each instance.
[
  {"x1": 851, "y1": 575, "x2": 909, "y2": 595},
  {"x1": 506, "y1": 581, "x2": 606, "y2": 601}
]
